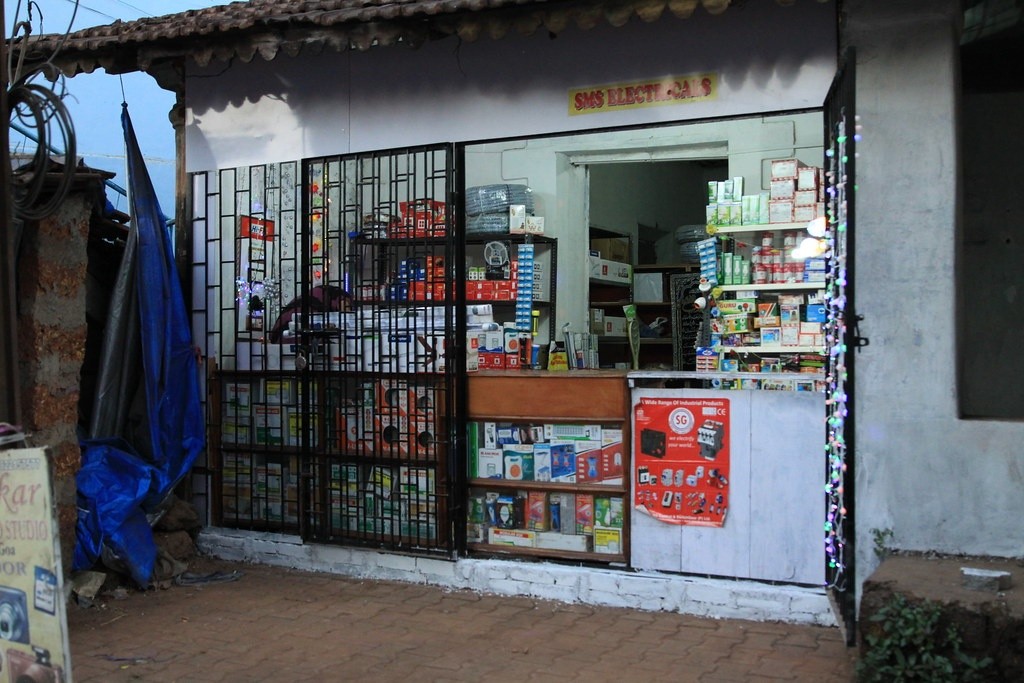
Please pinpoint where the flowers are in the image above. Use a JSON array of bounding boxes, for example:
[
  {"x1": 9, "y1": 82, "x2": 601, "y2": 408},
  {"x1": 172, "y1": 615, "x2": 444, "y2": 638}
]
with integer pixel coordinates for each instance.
[
  {"x1": 706, "y1": 223, "x2": 718, "y2": 235},
  {"x1": 711, "y1": 286, "x2": 724, "y2": 299},
  {"x1": 711, "y1": 306, "x2": 721, "y2": 317}
]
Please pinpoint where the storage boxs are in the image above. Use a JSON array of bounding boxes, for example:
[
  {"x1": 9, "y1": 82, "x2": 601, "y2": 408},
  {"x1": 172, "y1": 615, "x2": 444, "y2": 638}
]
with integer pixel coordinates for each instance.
[
  {"x1": 819, "y1": 185, "x2": 824, "y2": 202},
  {"x1": 797, "y1": 166, "x2": 819, "y2": 190},
  {"x1": 759, "y1": 193, "x2": 771, "y2": 225},
  {"x1": 742, "y1": 195, "x2": 750, "y2": 225},
  {"x1": 708, "y1": 180, "x2": 717, "y2": 205},
  {"x1": 771, "y1": 157, "x2": 809, "y2": 180},
  {"x1": 734, "y1": 177, "x2": 745, "y2": 201},
  {"x1": 717, "y1": 181, "x2": 725, "y2": 204},
  {"x1": 718, "y1": 204, "x2": 730, "y2": 227},
  {"x1": 770, "y1": 180, "x2": 798, "y2": 200},
  {"x1": 725, "y1": 179, "x2": 734, "y2": 203},
  {"x1": 795, "y1": 190, "x2": 818, "y2": 206},
  {"x1": 750, "y1": 194, "x2": 759, "y2": 225},
  {"x1": 819, "y1": 168, "x2": 825, "y2": 185},
  {"x1": 769, "y1": 199, "x2": 795, "y2": 224},
  {"x1": 590, "y1": 308, "x2": 605, "y2": 335},
  {"x1": 466, "y1": 491, "x2": 625, "y2": 555},
  {"x1": 697, "y1": 231, "x2": 826, "y2": 393},
  {"x1": 222, "y1": 199, "x2": 669, "y2": 554},
  {"x1": 706, "y1": 205, "x2": 717, "y2": 227},
  {"x1": 731, "y1": 203, "x2": 742, "y2": 226},
  {"x1": 794, "y1": 206, "x2": 815, "y2": 222},
  {"x1": 603, "y1": 317, "x2": 630, "y2": 337},
  {"x1": 815, "y1": 202, "x2": 825, "y2": 219},
  {"x1": 589, "y1": 255, "x2": 633, "y2": 285}
]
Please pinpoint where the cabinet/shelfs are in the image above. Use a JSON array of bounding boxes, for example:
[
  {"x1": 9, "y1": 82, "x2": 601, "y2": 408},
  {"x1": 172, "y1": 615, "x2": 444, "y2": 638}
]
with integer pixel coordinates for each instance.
[
  {"x1": 716, "y1": 222, "x2": 826, "y2": 390},
  {"x1": 212, "y1": 383, "x2": 447, "y2": 549},
  {"x1": 442, "y1": 370, "x2": 665, "y2": 564},
  {"x1": 353, "y1": 233, "x2": 558, "y2": 371},
  {"x1": 588, "y1": 222, "x2": 711, "y2": 390}
]
